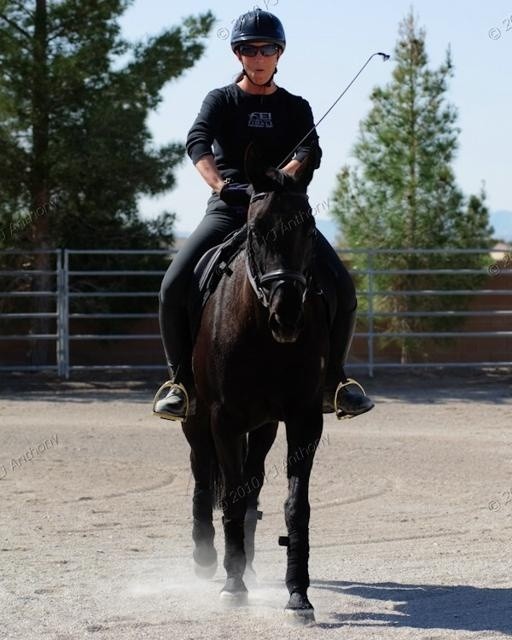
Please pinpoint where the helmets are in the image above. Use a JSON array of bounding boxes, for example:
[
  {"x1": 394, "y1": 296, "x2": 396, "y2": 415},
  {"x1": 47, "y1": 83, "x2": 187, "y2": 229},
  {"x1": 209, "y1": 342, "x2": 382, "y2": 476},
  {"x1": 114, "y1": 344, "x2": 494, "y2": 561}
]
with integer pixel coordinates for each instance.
[{"x1": 229, "y1": 8, "x2": 286, "y2": 53}]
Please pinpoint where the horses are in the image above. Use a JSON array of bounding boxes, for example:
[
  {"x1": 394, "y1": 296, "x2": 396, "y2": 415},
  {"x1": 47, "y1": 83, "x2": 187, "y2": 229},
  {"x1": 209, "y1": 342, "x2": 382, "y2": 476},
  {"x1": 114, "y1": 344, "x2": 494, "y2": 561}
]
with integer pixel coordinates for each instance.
[{"x1": 181, "y1": 143, "x2": 331, "y2": 626}]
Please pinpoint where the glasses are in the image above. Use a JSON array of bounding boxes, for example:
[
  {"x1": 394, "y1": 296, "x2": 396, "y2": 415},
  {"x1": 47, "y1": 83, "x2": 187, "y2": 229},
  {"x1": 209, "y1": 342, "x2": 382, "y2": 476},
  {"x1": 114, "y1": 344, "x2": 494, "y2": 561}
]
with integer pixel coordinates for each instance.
[{"x1": 238, "y1": 43, "x2": 279, "y2": 58}]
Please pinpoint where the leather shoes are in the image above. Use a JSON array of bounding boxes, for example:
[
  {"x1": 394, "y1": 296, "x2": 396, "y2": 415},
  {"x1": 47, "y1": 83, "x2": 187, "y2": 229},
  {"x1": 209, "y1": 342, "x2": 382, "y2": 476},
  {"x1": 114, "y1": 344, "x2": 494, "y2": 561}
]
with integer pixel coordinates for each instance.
[
  {"x1": 153, "y1": 365, "x2": 198, "y2": 418},
  {"x1": 321, "y1": 371, "x2": 375, "y2": 415}
]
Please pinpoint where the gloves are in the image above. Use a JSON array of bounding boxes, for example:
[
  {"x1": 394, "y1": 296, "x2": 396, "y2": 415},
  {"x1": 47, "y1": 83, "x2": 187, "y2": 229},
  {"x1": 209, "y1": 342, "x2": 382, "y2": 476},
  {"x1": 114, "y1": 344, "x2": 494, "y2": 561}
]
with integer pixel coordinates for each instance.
[
  {"x1": 252, "y1": 167, "x2": 291, "y2": 192},
  {"x1": 219, "y1": 182, "x2": 249, "y2": 207}
]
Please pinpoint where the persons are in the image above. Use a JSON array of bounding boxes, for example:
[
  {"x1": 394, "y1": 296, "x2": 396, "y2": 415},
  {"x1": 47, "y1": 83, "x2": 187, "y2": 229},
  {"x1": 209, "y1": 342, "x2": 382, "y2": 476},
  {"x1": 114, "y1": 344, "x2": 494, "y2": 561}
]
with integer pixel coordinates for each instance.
[{"x1": 154, "y1": 8, "x2": 374, "y2": 416}]
